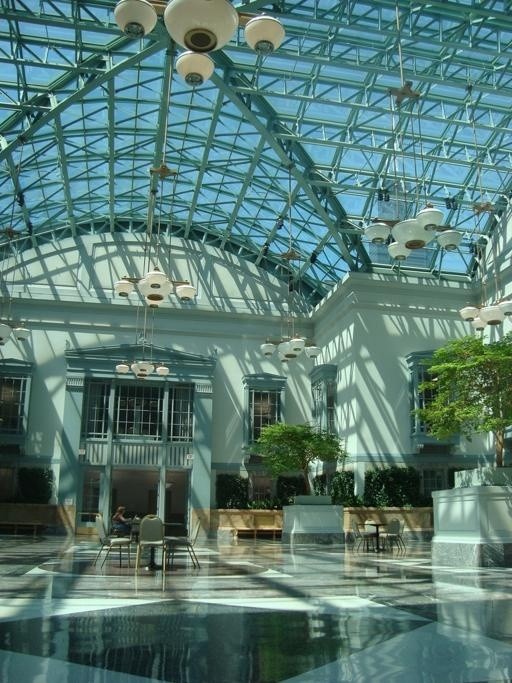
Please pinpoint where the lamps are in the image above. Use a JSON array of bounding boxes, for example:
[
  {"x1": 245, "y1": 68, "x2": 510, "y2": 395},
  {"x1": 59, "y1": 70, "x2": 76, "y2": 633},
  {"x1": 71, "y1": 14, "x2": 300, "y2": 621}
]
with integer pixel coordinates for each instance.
[
  {"x1": 113, "y1": 42, "x2": 198, "y2": 308},
  {"x1": 459, "y1": 212, "x2": 512, "y2": 330},
  {"x1": 116, "y1": 188, "x2": 170, "y2": 377},
  {"x1": 363, "y1": 42, "x2": 463, "y2": 260},
  {"x1": 114, "y1": 0, "x2": 286, "y2": 87},
  {"x1": 260, "y1": 165, "x2": 321, "y2": 362},
  {"x1": 0, "y1": 135, "x2": 32, "y2": 345}
]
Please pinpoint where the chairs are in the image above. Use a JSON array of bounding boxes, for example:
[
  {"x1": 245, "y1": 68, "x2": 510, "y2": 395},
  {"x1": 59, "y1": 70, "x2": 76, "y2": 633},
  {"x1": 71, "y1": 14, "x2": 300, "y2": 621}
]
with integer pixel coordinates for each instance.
[
  {"x1": 134, "y1": 514, "x2": 166, "y2": 594},
  {"x1": 351, "y1": 518, "x2": 406, "y2": 556},
  {"x1": 109, "y1": 514, "x2": 125, "y2": 538},
  {"x1": 164, "y1": 517, "x2": 201, "y2": 568},
  {"x1": 93, "y1": 513, "x2": 132, "y2": 569}
]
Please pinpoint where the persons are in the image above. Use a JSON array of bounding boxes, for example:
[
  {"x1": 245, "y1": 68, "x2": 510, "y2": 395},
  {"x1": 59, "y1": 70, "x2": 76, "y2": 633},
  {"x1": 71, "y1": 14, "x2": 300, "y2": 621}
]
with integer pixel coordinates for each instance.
[{"x1": 112, "y1": 505, "x2": 133, "y2": 539}]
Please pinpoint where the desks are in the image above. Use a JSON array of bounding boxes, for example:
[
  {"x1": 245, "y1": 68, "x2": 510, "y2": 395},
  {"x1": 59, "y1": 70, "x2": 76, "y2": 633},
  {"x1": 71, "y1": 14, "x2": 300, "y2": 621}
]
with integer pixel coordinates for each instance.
[{"x1": 125, "y1": 522, "x2": 186, "y2": 570}]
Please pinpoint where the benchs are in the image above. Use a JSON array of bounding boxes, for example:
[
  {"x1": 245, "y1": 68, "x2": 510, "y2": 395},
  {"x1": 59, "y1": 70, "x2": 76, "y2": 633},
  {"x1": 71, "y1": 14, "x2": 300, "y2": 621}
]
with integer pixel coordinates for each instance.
[{"x1": 0, "y1": 521, "x2": 45, "y2": 539}]
